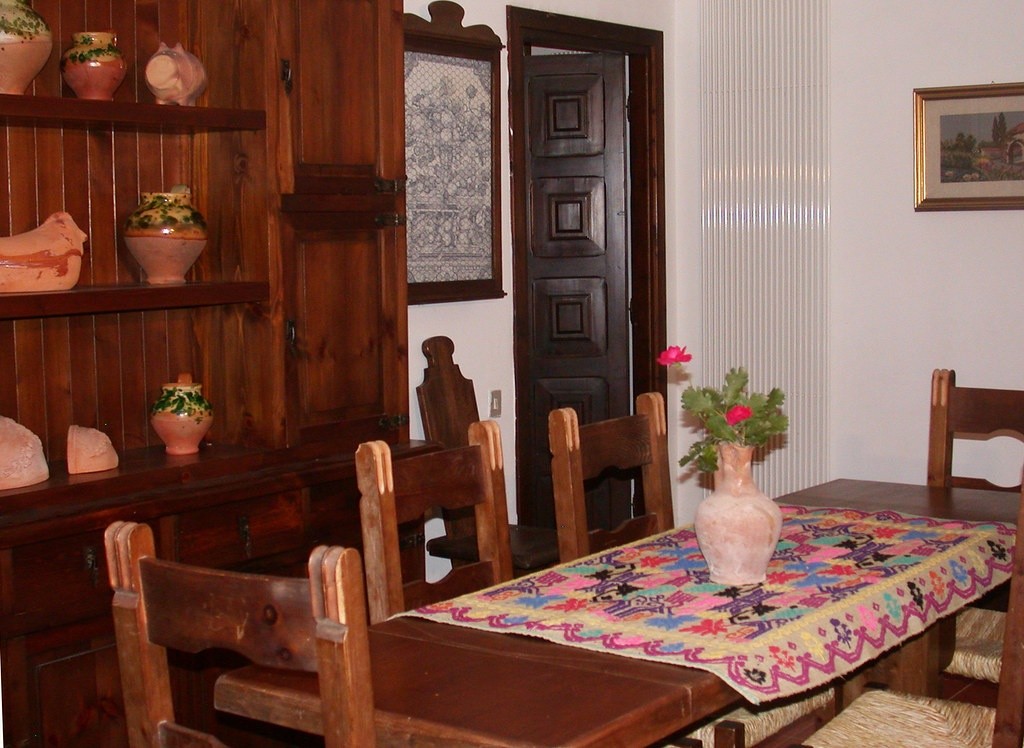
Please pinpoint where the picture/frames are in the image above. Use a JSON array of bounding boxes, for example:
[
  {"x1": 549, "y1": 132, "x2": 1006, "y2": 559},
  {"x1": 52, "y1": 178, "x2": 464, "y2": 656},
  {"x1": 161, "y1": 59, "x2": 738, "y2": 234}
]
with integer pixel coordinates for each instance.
[{"x1": 912, "y1": 82, "x2": 1024, "y2": 210}]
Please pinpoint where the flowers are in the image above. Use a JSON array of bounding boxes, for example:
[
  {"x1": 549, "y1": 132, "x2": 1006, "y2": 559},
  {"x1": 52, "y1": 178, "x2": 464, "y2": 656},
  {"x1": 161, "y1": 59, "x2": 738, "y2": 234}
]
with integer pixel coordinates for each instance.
[{"x1": 657, "y1": 344, "x2": 789, "y2": 473}]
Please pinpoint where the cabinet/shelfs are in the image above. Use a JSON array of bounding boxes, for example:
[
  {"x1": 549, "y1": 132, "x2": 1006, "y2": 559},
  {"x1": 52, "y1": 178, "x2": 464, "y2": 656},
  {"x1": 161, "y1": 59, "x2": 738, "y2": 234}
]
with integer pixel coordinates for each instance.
[{"x1": 0, "y1": 0, "x2": 444, "y2": 748}]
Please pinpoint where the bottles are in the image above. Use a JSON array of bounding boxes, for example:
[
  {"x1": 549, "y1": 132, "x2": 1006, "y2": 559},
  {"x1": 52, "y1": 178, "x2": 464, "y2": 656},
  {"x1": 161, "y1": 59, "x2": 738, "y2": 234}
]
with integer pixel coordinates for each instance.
[
  {"x1": 122, "y1": 190, "x2": 209, "y2": 286},
  {"x1": 0, "y1": 0, "x2": 54, "y2": 96},
  {"x1": 151, "y1": 381, "x2": 215, "y2": 456},
  {"x1": 59, "y1": 30, "x2": 127, "y2": 102}
]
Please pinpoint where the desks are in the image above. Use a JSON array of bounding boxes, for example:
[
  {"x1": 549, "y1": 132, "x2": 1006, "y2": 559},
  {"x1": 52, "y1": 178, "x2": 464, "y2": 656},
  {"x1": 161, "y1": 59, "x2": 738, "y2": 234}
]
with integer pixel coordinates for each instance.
[{"x1": 210, "y1": 477, "x2": 1024, "y2": 748}]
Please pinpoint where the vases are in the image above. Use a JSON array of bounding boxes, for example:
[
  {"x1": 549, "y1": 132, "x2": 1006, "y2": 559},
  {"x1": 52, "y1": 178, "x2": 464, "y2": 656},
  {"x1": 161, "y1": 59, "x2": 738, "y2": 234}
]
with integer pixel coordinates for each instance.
[
  {"x1": 124, "y1": 191, "x2": 209, "y2": 284},
  {"x1": 60, "y1": 32, "x2": 127, "y2": 101},
  {"x1": 694, "y1": 442, "x2": 783, "y2": 586},
  {"x1": 149, "y1": 373, "x2": 214, "y2": 456},
  {"x1": 0, "y1": 0, "x2": 53, "y2": 96}
]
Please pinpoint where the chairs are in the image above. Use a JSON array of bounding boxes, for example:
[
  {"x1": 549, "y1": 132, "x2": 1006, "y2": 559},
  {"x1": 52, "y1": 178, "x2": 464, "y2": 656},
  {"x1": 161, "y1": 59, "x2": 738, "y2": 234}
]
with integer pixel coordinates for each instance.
[
  {"x1": 549, "y1": 392, "x2": 863, "y2": 748},
  {"x1": 354, "y1": 421, "x2": 512, "y2": 625},
  {"x1": 104, "y1": 522, "x2": 375, "y2": 748},
  {"x1": 927, "y1": 368, "x2": 1024, "y2": 491},
  {"x1": 787, "y1": 500, "x2": 1023, "y2": 748}
]
